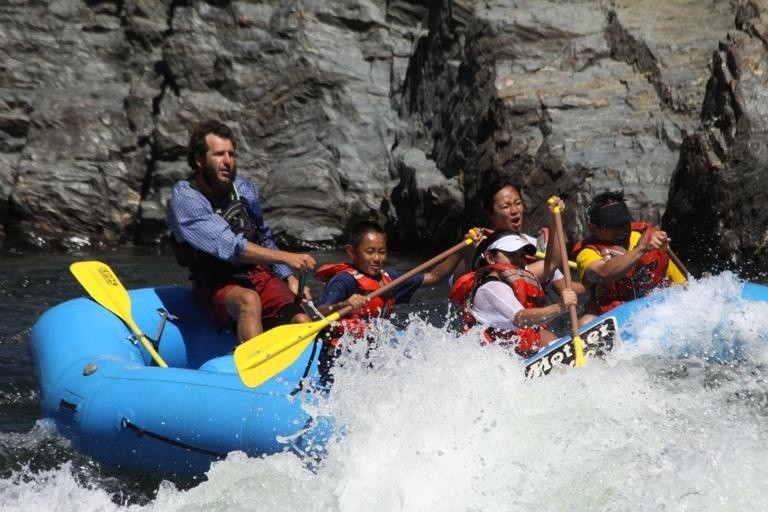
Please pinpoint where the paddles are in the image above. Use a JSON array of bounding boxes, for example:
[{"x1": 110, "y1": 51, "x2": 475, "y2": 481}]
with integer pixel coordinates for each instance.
[
  {"x1": 70, "y1": 260, "x2": 167, "y2": 368},
  {"x1": 233, "y1": 229, "x2": 479, "y2": 388}
]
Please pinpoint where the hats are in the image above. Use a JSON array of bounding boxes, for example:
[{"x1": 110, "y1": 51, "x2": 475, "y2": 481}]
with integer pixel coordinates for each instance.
[
  {"x1": 593, "y1": 199, "x2": 636, "y2": 227},
  {"x1": 486, "y1": 233, "x2": 536, "y2": 256}
]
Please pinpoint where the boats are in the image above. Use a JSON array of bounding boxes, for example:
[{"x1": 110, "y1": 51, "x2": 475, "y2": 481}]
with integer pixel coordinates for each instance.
[{"x1": 27, "y1": 276, "x2": 768, "y2": 479}]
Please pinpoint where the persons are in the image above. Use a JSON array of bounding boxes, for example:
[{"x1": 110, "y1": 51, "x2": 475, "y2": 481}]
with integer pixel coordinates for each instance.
[
  {"x1": 314, "y1": 221, "x2": 484, "y2": 393},
  {"x1": 449, "y1": 180, "x2": 594, "y2": 334},
  {"x1": 451, "y1": 194, "x2": 578, "y2": 363},
  {"x1": 571, "y1": 190, "x2": 696, "y2": 329},
  {"x1": 165, "y1": 118, "x2": 318, "y2": 348}
]
